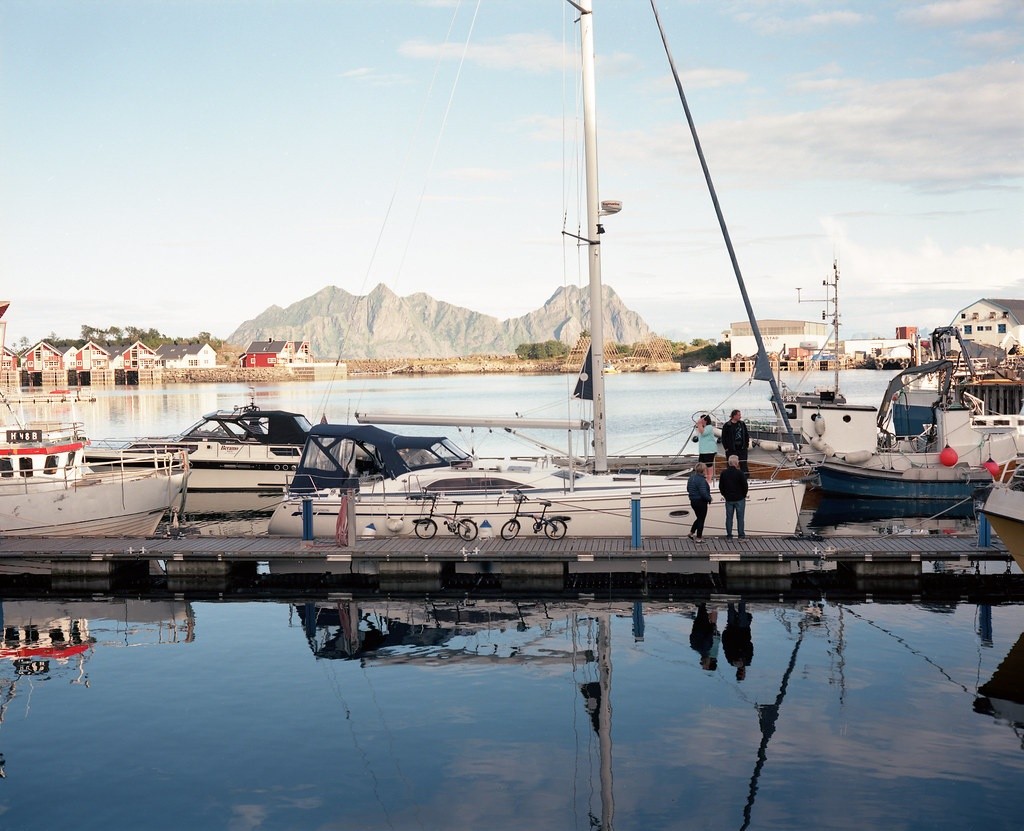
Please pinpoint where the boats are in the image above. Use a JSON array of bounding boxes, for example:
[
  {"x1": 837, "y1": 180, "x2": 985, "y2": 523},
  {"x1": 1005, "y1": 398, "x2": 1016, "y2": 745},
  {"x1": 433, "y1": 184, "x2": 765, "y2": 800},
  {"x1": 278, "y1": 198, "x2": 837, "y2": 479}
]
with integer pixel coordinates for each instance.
[
  {"x1": 690, "y1": 252, "x2": 878, "y2": 462},
  {"x1": 813, "y1": 356, "x2": 1024, "y2": 519},
  {"x1": 267, "y1": 422, "x2": 806, "y2": 539},
  {"x1": 982, "y1": 431, "x2": 1024, "y2": 575},
  {"x1": 899, "y1": 324, "x2": 1024, "y2": 395},
  {"x1": 82, "y1": 397, "x2": 427, "y2": 518},
  {"x1": 0, "y1": 387, "x2": 193, "y2": 536},
  {"x1": 0, "y1": 600, "x2": 197, "y2": 681}
]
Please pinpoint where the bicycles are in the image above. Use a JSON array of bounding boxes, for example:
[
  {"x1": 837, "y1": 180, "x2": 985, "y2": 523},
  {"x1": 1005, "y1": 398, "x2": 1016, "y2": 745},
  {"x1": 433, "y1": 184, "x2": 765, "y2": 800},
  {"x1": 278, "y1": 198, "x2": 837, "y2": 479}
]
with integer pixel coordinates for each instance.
[
  {"x1": 415, "y1": 492, "x2": 479, "y2": 542},
  {"x1": 500, "y1": 488, "x2": 571, "y2": 540}
]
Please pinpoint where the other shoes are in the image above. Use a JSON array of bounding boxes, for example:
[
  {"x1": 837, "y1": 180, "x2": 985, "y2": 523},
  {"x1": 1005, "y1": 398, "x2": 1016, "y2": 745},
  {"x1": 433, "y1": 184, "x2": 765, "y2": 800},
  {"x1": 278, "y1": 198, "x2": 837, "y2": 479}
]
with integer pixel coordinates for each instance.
[
  {"x1": 695, "y1": 539, "x2": 706, "y2": 544},
  {"x1": 738, "y1": 539, "x2": 750, "y2": 543},
  {"x1": 687, "y1": 533, "x2": 694, "y2": 541},
  {"x1": 724, "y1": 538, "x2": 733, "y2": 543}
]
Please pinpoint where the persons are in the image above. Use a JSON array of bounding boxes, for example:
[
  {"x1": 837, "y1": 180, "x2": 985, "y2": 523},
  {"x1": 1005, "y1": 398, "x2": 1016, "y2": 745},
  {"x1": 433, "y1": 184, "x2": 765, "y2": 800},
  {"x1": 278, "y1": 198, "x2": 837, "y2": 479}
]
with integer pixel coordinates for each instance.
[
  {"x1": 720, "y1": 410, "x2": 749, "y2": 479},
  {"x1": 697, "y1": 414, "x2": 718, "y2": 483},
  {"x1": 687, "y1": 463, "x2": 713, "y2": 543},
  {"x1": 689, "y1": 603, "x2": 754, "y2": 681},
  {"x1": 719, "y1": 455, "x2": 748, "y2": 538}
]
